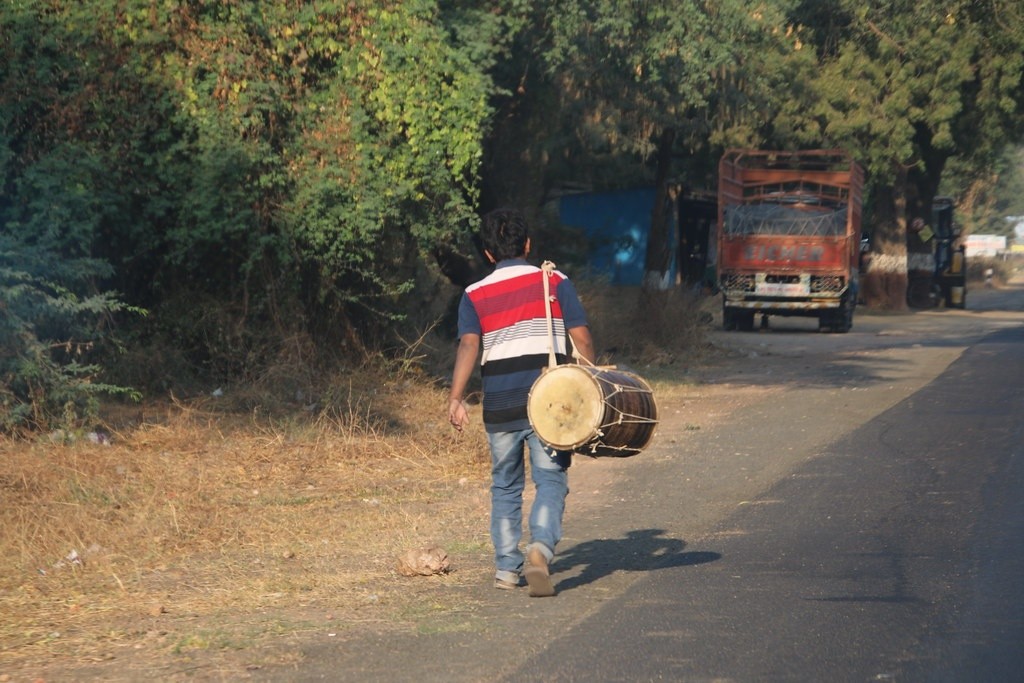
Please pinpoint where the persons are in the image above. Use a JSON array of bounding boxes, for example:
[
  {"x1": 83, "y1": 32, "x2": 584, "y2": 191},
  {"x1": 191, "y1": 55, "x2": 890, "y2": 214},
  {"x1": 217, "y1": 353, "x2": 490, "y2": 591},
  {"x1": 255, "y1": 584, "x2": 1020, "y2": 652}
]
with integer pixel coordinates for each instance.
[{"x1": 447, "y1": 207, "x2": 595, "y2": 597}]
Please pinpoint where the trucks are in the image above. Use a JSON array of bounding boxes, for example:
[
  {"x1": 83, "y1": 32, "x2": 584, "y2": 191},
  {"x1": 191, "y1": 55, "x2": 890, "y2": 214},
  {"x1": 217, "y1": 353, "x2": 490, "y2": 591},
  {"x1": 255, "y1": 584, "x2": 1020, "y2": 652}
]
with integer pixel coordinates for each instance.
[{"x1": 713, "y1": 146, "x2": 870, "y2": 334}]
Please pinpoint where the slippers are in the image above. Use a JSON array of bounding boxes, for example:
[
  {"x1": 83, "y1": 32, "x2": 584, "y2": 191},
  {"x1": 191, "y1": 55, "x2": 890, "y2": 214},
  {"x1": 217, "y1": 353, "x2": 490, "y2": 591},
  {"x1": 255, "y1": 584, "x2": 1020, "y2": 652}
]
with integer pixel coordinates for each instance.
[
  {"x1": 523, "y1": 566, "x2": 554, "y2": 596},
  {"x1": 494, "y1": 579, "x2": 516, "y2": 590}
]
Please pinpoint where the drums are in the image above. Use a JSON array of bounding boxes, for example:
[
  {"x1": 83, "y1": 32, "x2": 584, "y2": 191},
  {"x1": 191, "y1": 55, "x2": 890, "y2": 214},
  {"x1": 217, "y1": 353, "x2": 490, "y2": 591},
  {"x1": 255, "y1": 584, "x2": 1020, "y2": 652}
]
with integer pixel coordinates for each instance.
[{"x1": 525, "y1": 362, "x2": 661, "y2": 459}]
